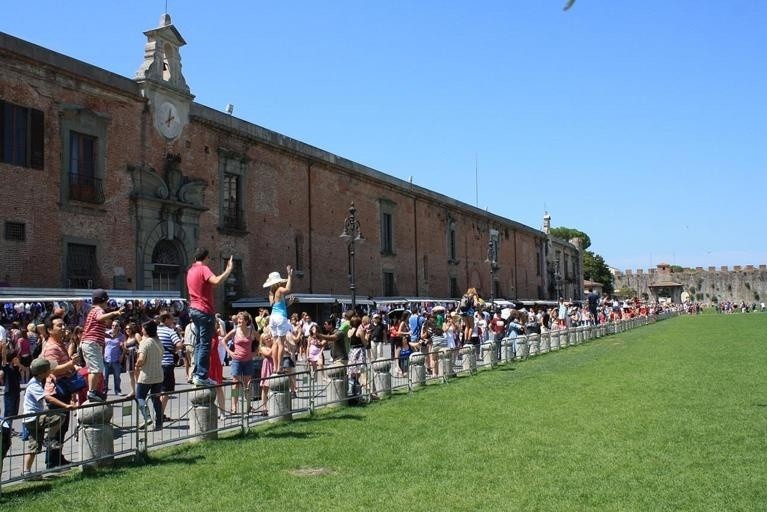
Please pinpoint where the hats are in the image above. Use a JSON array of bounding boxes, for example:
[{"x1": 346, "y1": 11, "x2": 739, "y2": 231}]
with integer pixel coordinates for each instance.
[
  {"x1": 372, "y1": 313, "x2": 381, "y2": 319},
  {"x1": 90, "y1": 287, "x2": 109, "y2": 303},
  {"x1": 29, "y1": 357, "x2": 51, "y2": 377},
  {"x1": 261, "y1": 271, "x2": 288, "y2": 288}
]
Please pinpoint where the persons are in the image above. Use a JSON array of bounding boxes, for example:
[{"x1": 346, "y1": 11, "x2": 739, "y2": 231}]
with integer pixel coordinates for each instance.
[
  {"x1": 185, "y1": 246, "x2": 234, "y2": 382},
  {"x1": 261, "y1": 265, "x2": 296, "y2": 376}
]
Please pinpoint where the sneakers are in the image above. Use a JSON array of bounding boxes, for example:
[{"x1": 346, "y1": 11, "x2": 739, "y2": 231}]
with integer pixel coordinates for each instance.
[
  {"x1": 114, "y1": 390, "x2": 127, "y2": 397},
  {"x1": 41, "y1": 436, "x2": 64, "y2": 451},
  {"x1": 86, "y1": 389, "x2": 107, "y2": 403},
  {"x1": 155, "y1": 414, "x2": 174, "y2": 422},
  {"x1": 191, "y1": 374, "x2": 219, "y2": 386},
  {"x1": 20, "y1": 470, "x2": 41, "y2": 481},
  {"x1": 217, "y1": 407, "x2": 270, "y2": 421},
  {"x1": 137, "y1": 418, "x2": 153, "y2": 431},
  {"x1": 11, "y1": 428, "x2": 23, "y2": 437}
]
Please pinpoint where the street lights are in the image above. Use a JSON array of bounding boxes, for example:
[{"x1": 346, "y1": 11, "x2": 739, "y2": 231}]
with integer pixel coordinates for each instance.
[
  {"x1": 485, "y1": 239, "x2": 501, "y2": 314},
  {"x1": 337, "y1": 199, "x2": 366, "y2": 310},
  {"x1": 552, "y1": 256, "x2": 563, "y2": 303}
]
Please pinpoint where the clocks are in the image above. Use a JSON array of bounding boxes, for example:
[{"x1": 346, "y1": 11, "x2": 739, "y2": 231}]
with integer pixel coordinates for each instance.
[{"x1": 156, "y1": 100, "x2": 182, "y2": 140}]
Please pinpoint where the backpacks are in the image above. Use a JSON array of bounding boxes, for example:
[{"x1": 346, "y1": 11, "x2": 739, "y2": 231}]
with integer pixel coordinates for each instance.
[{"x1": 458, "y1": 295, "x2": 471, "y2": 312}]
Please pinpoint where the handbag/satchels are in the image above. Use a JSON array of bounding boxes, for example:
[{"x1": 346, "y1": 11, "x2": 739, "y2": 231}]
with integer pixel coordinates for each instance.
[
  {"x1": 171, "y1": 352, "x2": 184, "y2": 367},
  {"x1": 63, "y1": 371, "x2": 87, "y2": 393}
]
